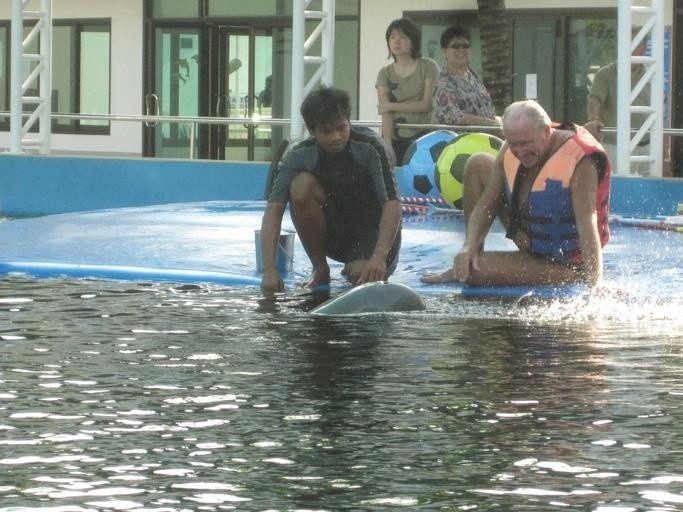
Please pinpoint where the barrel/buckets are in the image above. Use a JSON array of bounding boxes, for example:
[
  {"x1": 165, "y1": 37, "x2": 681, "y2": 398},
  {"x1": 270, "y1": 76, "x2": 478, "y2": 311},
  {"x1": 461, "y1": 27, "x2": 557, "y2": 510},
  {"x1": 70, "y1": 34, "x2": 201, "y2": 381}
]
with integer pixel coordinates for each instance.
[{"x1": 256, "y1": 229, "x2": 295, "y2": 272}]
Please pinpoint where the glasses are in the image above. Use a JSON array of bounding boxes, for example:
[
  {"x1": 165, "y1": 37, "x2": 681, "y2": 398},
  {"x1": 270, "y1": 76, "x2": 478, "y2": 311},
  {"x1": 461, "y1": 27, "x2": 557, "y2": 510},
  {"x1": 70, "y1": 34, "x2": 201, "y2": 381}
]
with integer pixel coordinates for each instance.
[{"x1": 450, "y1": 44, "x2": 471, "y2": 49}]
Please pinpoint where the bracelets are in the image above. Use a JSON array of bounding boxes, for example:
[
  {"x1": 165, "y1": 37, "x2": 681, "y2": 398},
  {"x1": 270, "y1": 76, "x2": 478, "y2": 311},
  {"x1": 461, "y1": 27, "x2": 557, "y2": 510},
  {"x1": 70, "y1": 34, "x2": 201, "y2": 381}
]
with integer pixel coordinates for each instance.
[{"x1": 261, "y1": 270, "x2": 279, "y2": 277}]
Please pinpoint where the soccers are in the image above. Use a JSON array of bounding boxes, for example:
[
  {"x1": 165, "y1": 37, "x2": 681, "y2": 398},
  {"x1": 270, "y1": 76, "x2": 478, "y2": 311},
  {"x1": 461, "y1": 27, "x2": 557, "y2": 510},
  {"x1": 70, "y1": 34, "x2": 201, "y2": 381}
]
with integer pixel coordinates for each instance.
[{"x1": 403, "y1": 130, "x2": 505, "y2": 210}]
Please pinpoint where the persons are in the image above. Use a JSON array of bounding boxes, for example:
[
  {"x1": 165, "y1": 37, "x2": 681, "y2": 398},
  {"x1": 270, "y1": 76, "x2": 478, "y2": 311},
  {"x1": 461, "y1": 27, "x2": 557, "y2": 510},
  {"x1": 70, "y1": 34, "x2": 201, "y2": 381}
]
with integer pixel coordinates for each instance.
[
  {"x1": 413, "y1": 97, "x2": 613, "y2": 293},
  {"x1": 427, "y1": 26, "x2": 500, "y2": 134},
  {"x1": 375, "y1": 16, "x2": 438, "y2": 169},
  {"x1": 581, "y1": 23, "x2": 670, "y2": 177},
  {"x1": 257, "y1": 84, "x2": 403, "y2": 295}
]
